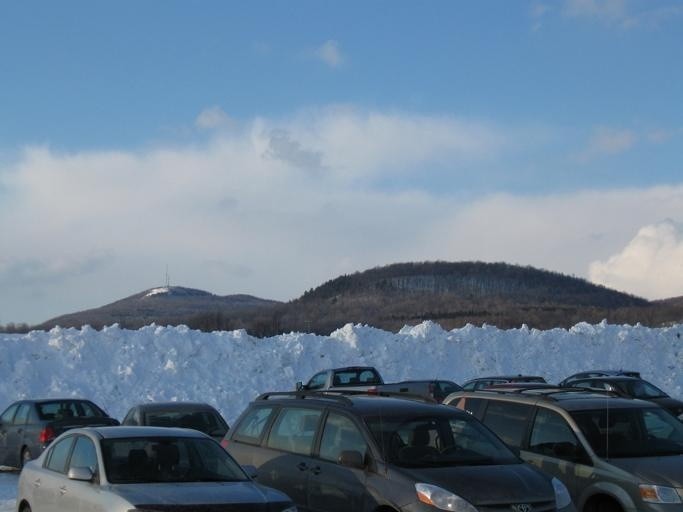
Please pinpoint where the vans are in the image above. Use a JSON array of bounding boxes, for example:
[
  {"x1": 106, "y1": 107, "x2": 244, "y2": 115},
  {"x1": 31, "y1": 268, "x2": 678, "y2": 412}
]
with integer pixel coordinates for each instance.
[
  {"x1": 224, "y1": 390, "x2": 578, "y2": 512},
  {"x1": 442, "y1": 390, "x2": 682, "y2": 511}
]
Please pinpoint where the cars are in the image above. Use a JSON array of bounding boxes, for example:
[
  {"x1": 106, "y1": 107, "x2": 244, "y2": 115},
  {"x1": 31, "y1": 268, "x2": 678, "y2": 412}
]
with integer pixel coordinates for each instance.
[{"x1": 19, "y1": 427, "x2": 295, "y2": 511}]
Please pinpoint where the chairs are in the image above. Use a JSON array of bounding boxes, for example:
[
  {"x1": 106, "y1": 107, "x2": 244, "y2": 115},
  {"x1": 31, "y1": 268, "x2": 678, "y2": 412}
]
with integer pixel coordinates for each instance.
[
  {"x1": 367, "y1": 425, "x2": 443, "y2": 467},
  {"x1": 291, "y1": 414, "x2": 326, "y2": 453}
]
